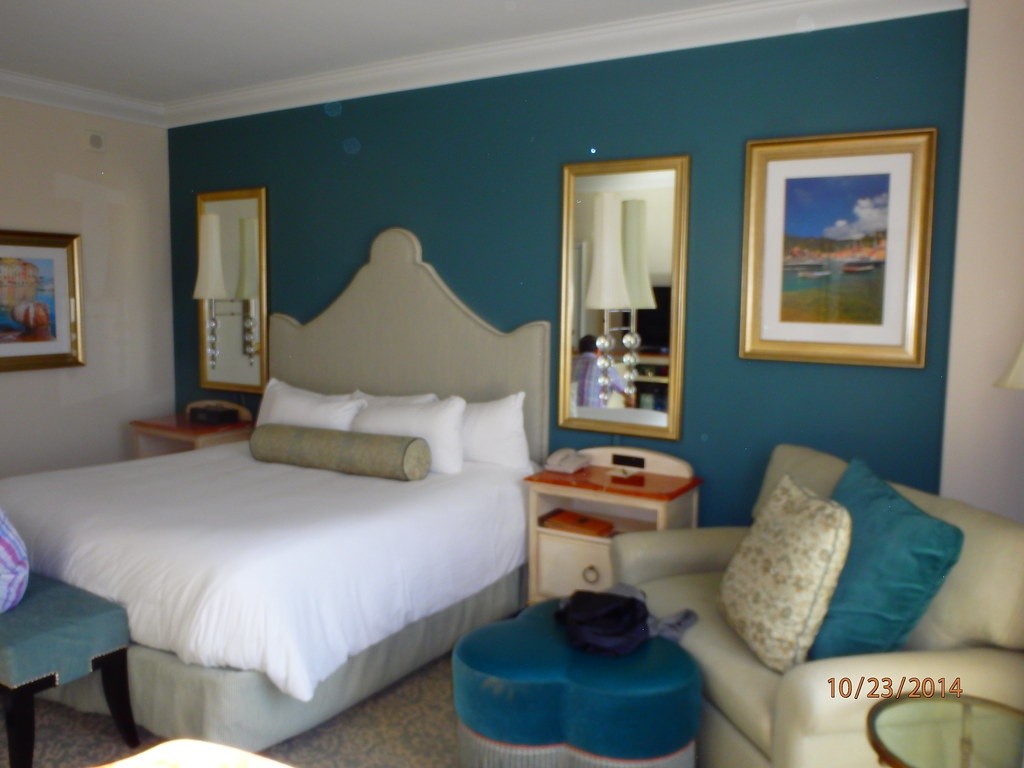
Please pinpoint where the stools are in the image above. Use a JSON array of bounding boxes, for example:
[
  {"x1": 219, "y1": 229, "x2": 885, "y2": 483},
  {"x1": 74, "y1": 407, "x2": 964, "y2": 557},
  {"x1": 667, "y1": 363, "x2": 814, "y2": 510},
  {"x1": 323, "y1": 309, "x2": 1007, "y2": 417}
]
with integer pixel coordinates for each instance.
[{"x1": 451, "y1": 598, "x2": 702, "y2": 768}]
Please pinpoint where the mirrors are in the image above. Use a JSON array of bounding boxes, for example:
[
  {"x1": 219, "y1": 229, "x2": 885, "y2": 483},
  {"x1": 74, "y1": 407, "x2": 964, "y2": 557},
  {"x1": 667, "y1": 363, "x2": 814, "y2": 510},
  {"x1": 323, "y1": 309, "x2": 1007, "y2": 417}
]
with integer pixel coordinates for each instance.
[
  {"x1": 557, "y1": 155, "x2": 692, "y2": 441},
  {"x1": 197, "y1": 188, "x2": 269, "y2": 394}
]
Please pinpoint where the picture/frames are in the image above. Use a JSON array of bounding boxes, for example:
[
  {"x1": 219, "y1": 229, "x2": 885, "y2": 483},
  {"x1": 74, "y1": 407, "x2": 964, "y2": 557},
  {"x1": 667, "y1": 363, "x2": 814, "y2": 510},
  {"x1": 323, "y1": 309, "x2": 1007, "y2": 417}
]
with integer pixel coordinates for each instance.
[
  {"x1": 0, "y1": 228, "x2": 86, "y2": 372},
  {"x1": 739, "y1": 127, "x2": 937, "y2": 368}
]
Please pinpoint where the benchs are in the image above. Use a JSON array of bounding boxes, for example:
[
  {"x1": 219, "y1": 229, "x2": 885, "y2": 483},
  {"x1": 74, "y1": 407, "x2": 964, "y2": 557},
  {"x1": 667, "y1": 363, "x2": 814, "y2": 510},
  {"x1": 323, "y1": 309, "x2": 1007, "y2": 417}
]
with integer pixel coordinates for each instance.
[{"x1": 0, "y1": 573, "x2": 139, "y2": 768}]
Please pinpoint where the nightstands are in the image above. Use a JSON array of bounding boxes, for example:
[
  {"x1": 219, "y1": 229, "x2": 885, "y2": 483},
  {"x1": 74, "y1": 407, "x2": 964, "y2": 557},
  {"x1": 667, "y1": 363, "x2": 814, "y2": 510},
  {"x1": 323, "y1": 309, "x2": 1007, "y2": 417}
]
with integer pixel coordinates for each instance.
[
  {"x1": 521, "y1": 446, "x2": 704, "y2": 607},
  {"x1": 131, "y1": 415, "x2": 255, "y2": 459}
]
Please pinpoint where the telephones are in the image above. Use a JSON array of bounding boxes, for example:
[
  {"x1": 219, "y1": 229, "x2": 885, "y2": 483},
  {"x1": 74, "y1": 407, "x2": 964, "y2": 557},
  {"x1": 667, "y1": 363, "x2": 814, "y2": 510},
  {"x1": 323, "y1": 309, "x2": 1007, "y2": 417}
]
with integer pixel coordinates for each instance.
[{"x1": 545, "y1": 447, "x2": 590, "y2": 474}]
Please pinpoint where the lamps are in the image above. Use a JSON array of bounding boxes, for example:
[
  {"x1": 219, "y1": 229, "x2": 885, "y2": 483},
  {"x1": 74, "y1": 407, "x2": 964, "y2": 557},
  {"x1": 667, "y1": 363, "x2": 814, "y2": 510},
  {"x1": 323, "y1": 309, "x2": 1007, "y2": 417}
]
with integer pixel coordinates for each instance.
[
  {"x1": 585, "y1": 192, "x2": 632, "y2": 405},
  {"x1": 231, "y1": 218, "x2": 260, "y2": 366},
  {"x1": 193, "y1": 214, "x2": 231, "y2": 370},
  {"x1": 619, "y1": 199, "x2": 657, "y2": 394}
]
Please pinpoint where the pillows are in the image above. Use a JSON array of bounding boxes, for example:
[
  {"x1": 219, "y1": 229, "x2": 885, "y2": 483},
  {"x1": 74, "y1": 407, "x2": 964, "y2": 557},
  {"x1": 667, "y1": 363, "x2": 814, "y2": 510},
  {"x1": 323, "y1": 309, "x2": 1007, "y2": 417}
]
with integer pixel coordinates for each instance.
[
  {"x1": 714, "y1": 473, "x2": 852, "y2": 673},
  {"x1": 808, "y1": 458, "x2": 963, "y2": 662},
  {"x1": 253, "y1": 376, "x2": 532, "y2": 481}
]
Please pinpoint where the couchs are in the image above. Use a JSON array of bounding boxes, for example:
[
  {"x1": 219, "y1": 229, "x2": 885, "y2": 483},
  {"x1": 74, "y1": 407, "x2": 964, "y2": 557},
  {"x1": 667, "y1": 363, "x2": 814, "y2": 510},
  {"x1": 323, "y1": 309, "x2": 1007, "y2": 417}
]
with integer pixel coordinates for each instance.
[{"x1": 608, "y1": 443, "x2": 1024, "y2": 768}]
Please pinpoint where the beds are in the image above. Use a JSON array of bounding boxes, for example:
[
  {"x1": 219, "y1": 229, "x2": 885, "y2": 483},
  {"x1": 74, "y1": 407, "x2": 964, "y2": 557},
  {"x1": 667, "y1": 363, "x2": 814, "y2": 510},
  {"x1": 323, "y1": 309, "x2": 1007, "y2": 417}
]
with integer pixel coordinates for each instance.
[{"x1": 0, "y1": 228, "x2": 552, "y2": 753}]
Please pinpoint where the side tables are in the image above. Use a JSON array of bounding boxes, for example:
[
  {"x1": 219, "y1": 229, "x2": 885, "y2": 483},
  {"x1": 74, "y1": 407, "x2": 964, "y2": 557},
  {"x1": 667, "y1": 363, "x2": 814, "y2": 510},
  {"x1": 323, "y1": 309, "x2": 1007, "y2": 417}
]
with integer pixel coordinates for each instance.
[{"x1": 866, "y1": 692, "x2": 1024, "y2": 768}]
